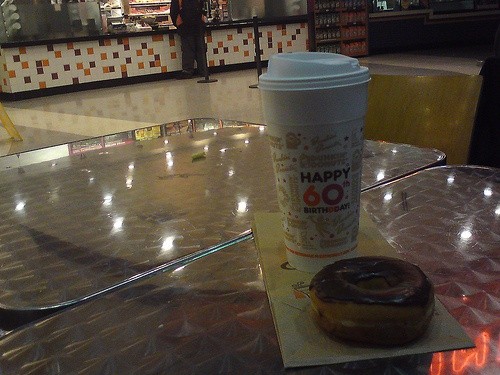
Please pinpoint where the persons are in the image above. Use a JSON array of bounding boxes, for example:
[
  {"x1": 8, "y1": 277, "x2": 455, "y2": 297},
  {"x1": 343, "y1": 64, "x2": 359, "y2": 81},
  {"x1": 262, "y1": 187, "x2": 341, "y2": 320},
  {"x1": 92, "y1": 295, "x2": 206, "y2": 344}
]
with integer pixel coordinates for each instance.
[{"x1": 170, "y1": 0, "x2": 208, "y2": 80}]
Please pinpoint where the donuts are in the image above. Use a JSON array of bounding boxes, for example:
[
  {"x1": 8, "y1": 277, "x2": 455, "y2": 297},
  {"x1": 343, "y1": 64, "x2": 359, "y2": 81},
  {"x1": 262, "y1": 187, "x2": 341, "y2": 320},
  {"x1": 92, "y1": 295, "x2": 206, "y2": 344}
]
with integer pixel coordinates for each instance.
[{"x1": 309, "y1": 255, "x2": 436, "y2": 349}]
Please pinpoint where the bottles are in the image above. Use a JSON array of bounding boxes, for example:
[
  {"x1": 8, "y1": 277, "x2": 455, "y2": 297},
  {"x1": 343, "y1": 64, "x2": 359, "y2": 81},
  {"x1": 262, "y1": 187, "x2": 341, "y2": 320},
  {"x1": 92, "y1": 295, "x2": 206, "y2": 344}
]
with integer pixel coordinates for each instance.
[{"x1": 314, "y1": 0, "x2": 368, "y2": 55}]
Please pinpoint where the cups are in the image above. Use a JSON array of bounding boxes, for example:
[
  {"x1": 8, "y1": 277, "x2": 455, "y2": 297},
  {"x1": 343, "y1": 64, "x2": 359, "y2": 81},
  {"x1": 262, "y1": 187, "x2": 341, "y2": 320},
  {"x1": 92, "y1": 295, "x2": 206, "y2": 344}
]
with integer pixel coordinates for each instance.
[
  {"x1": 1, "y1": 0, "x2": 22, "y2": 35},
  {"x1": 258, "y1": 52, "x2": 371, "y2": 271}
]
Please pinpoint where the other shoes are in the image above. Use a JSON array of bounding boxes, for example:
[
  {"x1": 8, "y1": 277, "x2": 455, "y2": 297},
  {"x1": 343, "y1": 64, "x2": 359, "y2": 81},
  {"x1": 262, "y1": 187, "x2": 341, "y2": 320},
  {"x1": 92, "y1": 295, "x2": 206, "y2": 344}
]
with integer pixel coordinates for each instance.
[
  {"x1": 199, "y1": 70, "x2": 208, "y2": 76},
  {"x1": 176, "y1": 71, "x2": 192, "y2": 79}
]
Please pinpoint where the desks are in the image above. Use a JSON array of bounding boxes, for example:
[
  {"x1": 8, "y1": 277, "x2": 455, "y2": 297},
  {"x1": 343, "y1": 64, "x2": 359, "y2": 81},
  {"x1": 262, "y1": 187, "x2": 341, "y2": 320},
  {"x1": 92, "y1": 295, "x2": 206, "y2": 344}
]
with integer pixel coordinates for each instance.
[{"x1": 1, "y1": 118, "x2": 500, "y2": 375}]
